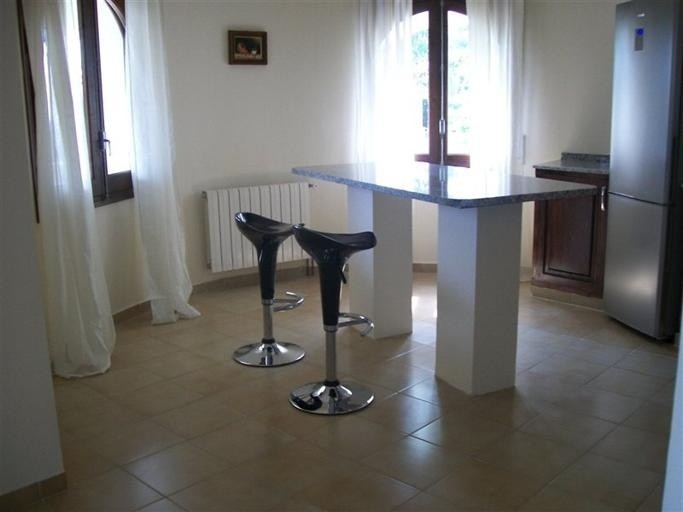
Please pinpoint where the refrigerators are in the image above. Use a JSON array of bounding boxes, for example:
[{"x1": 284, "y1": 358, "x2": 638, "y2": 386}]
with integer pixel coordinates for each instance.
[{"x1": 604, "y1": 2, "x2": 682, "y2": 342}]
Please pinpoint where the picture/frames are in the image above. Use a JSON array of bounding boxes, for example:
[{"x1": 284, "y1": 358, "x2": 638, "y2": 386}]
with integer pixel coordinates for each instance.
[{"x1": 228, "y1": 29, "x2": 267, "y2": 66}]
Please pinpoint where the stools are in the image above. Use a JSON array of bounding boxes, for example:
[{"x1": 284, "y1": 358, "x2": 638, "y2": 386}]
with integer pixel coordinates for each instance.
[
  {"x1": 288, "y1": 224, "x2": 375, "y2": 414},
  {"x1": 234, "y1": 212, "x2": 304, "y2": 368}
]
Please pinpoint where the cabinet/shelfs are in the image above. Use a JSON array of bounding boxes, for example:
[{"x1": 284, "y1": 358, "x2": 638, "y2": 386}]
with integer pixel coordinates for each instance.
[{"x1": 530, "y1": 168, "x2": 608, "y2": 300}]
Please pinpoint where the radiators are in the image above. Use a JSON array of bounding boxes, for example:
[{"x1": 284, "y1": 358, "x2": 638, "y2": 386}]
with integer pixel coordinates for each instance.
[{"x1": 201, "y1": 182, "x2": 318, "y2": 274}]
